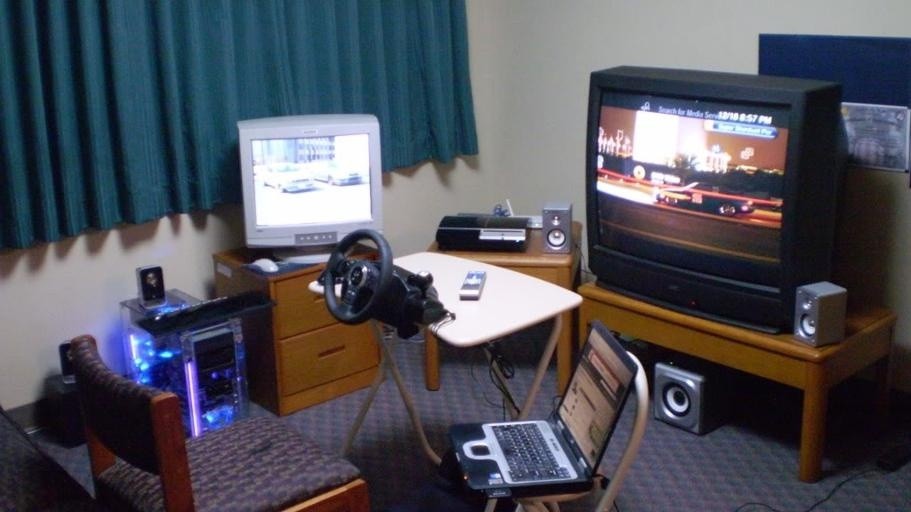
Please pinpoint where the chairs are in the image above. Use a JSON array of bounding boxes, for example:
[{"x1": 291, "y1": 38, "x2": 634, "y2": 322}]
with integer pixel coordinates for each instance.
[
  {"x1": 486, "y1": 350, "x2": 649, "y2": 512},
  {"x1": 71, "y1": 332, "x2": 371, "y2": 511}
]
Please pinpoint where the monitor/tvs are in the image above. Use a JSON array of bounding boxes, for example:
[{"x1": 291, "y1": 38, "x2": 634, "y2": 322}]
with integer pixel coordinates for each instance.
[
  {"x1": 235, "y1": 112, "x2": 384, "y2": 264},
  {"x1": 585, "y1": 64, "x2": 849, "y2": 336}
]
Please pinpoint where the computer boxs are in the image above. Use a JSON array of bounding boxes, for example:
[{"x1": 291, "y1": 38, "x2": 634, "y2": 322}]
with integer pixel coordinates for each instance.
[{"x1": 120, "y1": 289, "x2": 250, "y2": 437}]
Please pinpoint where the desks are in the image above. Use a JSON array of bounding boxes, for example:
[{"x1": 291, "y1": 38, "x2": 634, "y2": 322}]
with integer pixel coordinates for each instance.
[
  {"x1": 343, "y1": 249, "x2": 584, "y2": 460},
  {"x1": 425, "y1": 222, "x2": 583, "y2": 395},
  {"x1": 577, "y1": 283, "x2": 899, "y2": 483}
]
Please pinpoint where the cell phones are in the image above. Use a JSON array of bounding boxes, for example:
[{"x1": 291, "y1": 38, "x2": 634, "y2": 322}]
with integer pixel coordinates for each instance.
[{"x1": 460, "y1": 270, "x2": 487, "y2": 300}]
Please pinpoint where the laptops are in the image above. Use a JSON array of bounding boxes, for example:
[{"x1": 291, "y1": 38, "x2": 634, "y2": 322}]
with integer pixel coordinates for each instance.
[{"x1": 450, "y1": 318, "x2": 638, "y2": 497}]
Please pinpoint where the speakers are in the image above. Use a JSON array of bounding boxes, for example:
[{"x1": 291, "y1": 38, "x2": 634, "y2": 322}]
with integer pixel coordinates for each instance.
[
  {"x1": 793, "y1": 280, "x2": 848, "y2": 347},
  {"x1": 543, "y1": 203, "x2": 572, "y2": 254},
  {"x1": 136, "y1": 264, "x2": 168, "y2": 310},
  {"x1": 59, "y1": 343, "x2": 76, "y2": 384},
  {"x1": 652, "y1": 361, "x2": 733, "y2": 436}
]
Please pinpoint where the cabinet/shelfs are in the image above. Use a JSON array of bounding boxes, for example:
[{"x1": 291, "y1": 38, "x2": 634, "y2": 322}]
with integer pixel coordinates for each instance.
[{"x1": 211, "y1": 238, "x2": 386, "y2": 418}]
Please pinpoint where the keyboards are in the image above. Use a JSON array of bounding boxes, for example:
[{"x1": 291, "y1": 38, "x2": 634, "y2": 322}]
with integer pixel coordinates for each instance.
[{"x1": 136, "y1": 290, "x2": 278, "y2": 338}]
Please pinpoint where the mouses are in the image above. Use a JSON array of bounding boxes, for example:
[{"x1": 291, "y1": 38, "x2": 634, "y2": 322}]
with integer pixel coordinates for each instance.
[{"x1": 248, "y1": 258, "x2": 280, "y2": 273}]
